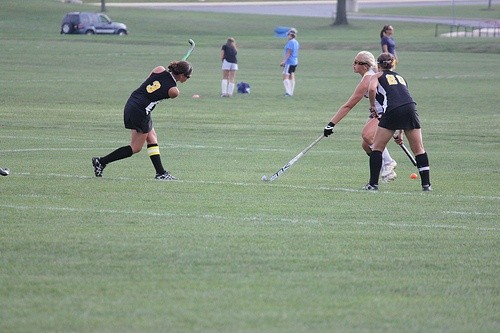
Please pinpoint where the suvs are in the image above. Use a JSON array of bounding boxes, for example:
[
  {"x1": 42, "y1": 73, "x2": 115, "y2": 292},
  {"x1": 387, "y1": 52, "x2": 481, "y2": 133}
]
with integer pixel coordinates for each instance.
[{"x1": 61, "y1": 11, "x2": 128, "y2": 37}]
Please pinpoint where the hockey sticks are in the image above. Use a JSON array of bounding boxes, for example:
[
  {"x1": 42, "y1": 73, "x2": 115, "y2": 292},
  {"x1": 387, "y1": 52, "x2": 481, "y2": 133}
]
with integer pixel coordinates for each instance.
[
  {"x1": 369, "y1": 107, "x2": 417, "y2": 166},
  {"x1": 261, "y1": 134, "x2": 324, "y2": 183},
  {"x1": 182, "y1": 39, "x2": 196, "y2": 61},
  {"x1": 0, "y1": 168, "x2": 11, "y2": 176}
]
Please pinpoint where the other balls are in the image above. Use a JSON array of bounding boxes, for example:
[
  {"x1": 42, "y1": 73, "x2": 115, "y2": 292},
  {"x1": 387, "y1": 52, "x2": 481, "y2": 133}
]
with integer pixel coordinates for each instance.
[{"x1": 411, "y1": 173, "x2": 417, "y2": 179}]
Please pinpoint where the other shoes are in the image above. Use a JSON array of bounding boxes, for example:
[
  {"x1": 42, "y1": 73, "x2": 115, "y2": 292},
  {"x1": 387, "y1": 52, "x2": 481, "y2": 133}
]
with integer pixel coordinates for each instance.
[
  {"x1": 421, "y1": 184, "x2": 432, "y2": 191},
  {"x1": 362, "y1": 182, "x2": 377, "y2": 191}
]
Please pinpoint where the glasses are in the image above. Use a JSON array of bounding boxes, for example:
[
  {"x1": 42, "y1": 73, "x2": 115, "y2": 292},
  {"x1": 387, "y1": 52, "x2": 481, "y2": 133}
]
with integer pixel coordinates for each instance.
[
  {"x1": 386, "y1": 27, "x2": 393, "y2": 31},
  {"x1": 183, "y1": 74, "x2": 191, "y2": 79},
  {"x1": 354, "y1": 60, "x2": 370, "y2": 66}
]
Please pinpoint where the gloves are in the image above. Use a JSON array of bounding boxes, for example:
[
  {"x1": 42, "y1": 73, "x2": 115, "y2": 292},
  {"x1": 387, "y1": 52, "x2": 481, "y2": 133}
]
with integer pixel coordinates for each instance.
[{"x1": 323, "y1": 121, "x2": 334, "y2": 137}]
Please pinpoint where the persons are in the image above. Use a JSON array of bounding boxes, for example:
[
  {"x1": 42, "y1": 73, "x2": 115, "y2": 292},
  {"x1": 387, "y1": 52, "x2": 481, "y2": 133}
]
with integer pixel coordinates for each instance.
[
  {"x1": 361, "y1": 53, "x2": 432, "y2": 192},
  {"x1": 92, "y1": 61, "x2": 192, "y2": 180},
  {"x1": 220, "y1": 39, "x2": 238, "y2": 97},
  {"x1": 280, "y1": 28, "x2": 299, "y2": 97},
  {"x1": 324, "y1": 51, "x2": 397, "y2": 183},
  {"x1": 380, "y1": 25, "x2": 398, "y2": 70}
]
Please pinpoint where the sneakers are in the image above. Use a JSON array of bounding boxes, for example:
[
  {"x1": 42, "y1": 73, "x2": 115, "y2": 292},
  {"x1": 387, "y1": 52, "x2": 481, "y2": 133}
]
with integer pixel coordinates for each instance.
[
  {"x1": 155, "y1": 170, "x2": 178, "y2": 180},
  {"x1": 92, "y1": 156, "x2": 106, "y2": 177},
  {"x1": 379, "y1": 170, "x2": 396, "y2": 183},
  {"x1": 381, "y1": 158, "x2": 398, "y2": 177}
]
